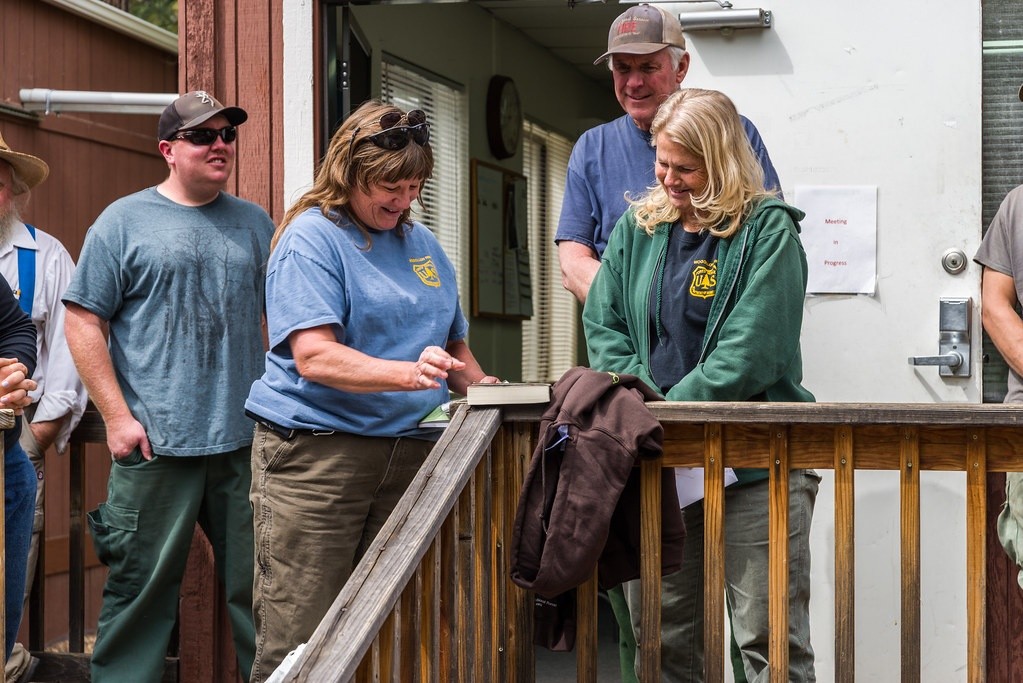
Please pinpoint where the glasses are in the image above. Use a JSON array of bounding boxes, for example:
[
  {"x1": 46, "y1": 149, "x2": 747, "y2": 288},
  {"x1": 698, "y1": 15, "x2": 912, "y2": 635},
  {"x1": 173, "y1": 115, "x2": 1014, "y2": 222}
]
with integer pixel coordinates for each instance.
[
  {"x1": 348, "y1": 109, "x2": 426, "y2": 161},
  {"x1": 170, "y1": 126, "x2": 236, "y2": 145},
  {"x1": 354, "y1": 123, "x2": 430, "y2": 151}
]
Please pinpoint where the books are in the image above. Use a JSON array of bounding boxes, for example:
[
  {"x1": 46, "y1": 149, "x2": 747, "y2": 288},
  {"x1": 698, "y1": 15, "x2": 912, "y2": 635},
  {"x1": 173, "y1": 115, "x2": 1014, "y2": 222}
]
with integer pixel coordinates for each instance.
[{"x1": 419, "y1": 380, "x2": 550, "y2": 428}]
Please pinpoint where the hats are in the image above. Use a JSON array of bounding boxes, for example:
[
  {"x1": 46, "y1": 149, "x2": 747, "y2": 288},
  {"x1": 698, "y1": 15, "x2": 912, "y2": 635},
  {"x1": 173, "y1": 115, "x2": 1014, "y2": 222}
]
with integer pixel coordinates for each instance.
[
  {"x1": 593, "y1": 4, "x2": 687, "y2": 66},
  {"x1": 0, "y1": 131, "x2": 49, "y2": 196},
  {"x1": 158, "y1": 91, "x2": 248, "y2": 143}
]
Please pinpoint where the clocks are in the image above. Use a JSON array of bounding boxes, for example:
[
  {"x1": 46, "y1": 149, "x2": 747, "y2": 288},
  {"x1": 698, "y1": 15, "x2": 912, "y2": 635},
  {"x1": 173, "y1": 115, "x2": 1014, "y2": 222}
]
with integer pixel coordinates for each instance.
[{"x1": 485, "y1": 75, "x2": 521, "y2": 159}]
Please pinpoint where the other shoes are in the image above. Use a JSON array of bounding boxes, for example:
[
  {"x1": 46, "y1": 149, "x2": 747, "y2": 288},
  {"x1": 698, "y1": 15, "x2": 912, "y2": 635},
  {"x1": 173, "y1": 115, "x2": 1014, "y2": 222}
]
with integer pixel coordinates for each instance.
[{"x1": 6, "y1": 643, "x2": 40, "y2": 683}]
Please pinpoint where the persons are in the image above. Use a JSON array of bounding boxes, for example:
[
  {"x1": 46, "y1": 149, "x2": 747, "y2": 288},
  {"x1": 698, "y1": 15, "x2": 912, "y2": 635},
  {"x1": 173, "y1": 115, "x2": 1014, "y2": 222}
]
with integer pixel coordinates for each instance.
[
  {"x1": 60, "y1": 90, "x2": 277, "y2": 683},
  {"x1": 0, "y1": 130, "x2": 89, "y2": 683},
  {"x1": 244, "y1": 102, "x2": 502, "y2": 683},
  {"x1": 583, "y1": 89, "x2": 822, "y2": 683},
  {"x1": 552, "y1": 3, "x2": 787, "y2": 308},
  {"x1": 973, "y1": 184, "x2": 1023, "y2": 590}
]
